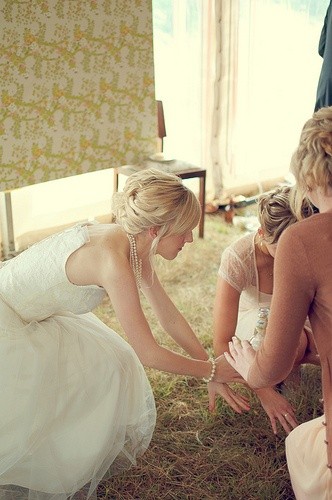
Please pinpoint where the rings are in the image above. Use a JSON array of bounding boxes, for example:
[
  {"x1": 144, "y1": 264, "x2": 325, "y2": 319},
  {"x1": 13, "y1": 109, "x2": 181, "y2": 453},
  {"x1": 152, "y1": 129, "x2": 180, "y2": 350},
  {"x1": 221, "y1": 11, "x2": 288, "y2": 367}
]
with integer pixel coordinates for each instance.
[
  {"x1": 232, "y1": 391, "x2": 237, "y2": 396},
  {"x1": 283, "y1": 412, "x2": 290, "y2": 418}
]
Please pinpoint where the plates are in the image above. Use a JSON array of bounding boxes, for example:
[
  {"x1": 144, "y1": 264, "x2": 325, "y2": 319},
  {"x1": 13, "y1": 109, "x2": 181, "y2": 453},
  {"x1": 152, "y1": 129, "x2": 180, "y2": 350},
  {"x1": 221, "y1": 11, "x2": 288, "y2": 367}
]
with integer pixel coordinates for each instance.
[{"x1": 150, "y1": 155, "x2": 175, "y2": 161}]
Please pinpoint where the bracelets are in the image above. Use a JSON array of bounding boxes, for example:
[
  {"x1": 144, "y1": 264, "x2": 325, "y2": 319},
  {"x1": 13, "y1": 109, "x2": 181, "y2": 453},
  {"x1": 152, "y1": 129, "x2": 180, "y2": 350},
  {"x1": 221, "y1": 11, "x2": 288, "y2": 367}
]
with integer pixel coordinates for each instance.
[{"x1": 203, "y1": 360, "x2": 217, "y2": 386}]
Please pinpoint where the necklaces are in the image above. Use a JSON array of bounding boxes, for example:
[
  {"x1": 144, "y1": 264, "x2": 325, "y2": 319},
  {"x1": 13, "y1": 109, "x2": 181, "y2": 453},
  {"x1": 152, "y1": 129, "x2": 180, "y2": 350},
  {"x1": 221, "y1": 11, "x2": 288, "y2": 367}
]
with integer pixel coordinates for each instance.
[
  {"x1": 126, "y1": 232, "x2": 143, "y2": 294},
  {"x1": 259, "y1": 235, "x2": 273, "y2": 282}
]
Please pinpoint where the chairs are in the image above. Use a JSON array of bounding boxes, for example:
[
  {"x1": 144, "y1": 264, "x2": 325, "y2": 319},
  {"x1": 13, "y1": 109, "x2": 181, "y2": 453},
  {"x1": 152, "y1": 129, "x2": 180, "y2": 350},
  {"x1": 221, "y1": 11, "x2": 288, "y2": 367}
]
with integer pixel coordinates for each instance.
[{"x1": 112, "y1": 99, "x2": 207, "y2": 239}]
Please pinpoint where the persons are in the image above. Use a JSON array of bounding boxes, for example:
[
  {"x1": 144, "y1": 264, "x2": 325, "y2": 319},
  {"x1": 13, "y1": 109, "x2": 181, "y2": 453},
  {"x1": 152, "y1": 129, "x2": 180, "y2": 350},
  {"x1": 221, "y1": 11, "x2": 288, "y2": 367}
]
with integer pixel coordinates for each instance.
[
  {"x1": 224, "y1": 107, "x2": 332, "y2": 500},
  {"x1": 213, "y1": 184, "x2": 327, "y2": 435},
  {"x1": 314, "y1": 0, "x2": 332, "y2": 112},
  {"x1": 0, "y1": 168, "x2": 251, "y2": 500}
]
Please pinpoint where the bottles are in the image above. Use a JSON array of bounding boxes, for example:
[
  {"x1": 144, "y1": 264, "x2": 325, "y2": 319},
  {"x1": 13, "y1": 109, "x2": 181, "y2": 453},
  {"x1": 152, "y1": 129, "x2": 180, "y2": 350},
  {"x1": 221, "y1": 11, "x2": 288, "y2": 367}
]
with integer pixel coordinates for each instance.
[
  {"x1": 225, "y1": 199, "x2": 235, "y2": 225},
  {"x1": 249, "y1": 307, "x2": 271, "y2": 353}
]
